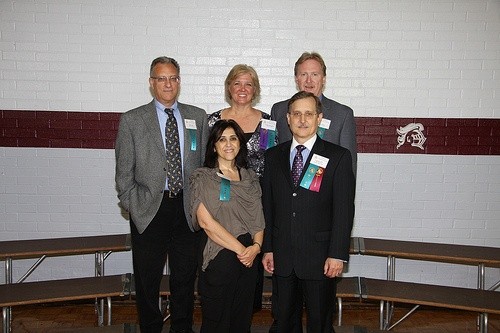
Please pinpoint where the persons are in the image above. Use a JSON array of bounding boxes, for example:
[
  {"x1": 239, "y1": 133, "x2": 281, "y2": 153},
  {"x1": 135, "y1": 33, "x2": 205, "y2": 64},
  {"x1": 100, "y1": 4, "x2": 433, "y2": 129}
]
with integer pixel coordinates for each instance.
[
  {"x1": 205, "y1": 64, "x2": 277, "y2": 185},
  {"x1": 114, "y1": 56, "x2": 210, "y2": 333},
  {"x1": 188, "y1": 118, "x2": 266, "y2": 333},
  {"x1": 270, "y1": 52, "x2": 357, "y2": 179},
  {"x1": 261, "y1": 91, "x2": 356, "y2": 333}
]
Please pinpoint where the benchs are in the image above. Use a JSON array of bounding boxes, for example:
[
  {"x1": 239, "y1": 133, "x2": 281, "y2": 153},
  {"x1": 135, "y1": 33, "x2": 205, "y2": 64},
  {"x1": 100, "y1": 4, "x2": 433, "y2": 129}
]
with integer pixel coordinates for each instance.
[{"x1": 0, "y1": 234, "x2": 500, "y2": 333}]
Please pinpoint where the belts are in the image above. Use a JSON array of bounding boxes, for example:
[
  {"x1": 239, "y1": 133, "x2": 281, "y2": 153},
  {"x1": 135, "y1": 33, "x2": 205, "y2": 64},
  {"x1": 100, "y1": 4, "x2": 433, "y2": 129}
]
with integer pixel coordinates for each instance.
[{"x1": 163, "y1": 191, "x2": 183, "y2": 196}]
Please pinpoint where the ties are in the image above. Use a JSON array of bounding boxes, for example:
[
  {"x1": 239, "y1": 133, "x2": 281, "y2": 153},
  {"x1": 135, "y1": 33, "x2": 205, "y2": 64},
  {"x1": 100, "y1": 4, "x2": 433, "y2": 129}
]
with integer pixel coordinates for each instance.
[
  {"x1": 292, "y1": 145, "x2": 306, "y2": 187},
  {"x1": 164, "y1": 108, "x2": 183, "y2": 195}
]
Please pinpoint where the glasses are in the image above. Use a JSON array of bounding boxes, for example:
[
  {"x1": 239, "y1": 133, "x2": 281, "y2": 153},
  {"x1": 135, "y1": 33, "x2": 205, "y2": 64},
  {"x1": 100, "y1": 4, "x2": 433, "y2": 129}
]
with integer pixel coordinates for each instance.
[
  {"x1": 290, "y1": 111, "x2": 319, "y2": 118},
  {"x1": 150, "y1": 76, "x2": 179, "y2": 82}
]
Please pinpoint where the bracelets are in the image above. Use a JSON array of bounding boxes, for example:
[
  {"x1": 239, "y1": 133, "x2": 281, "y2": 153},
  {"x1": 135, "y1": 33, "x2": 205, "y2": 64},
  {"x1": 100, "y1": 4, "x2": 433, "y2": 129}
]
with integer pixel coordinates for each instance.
[{"x1": 252, "y1": 242, "x2": 261, "y2": 253}]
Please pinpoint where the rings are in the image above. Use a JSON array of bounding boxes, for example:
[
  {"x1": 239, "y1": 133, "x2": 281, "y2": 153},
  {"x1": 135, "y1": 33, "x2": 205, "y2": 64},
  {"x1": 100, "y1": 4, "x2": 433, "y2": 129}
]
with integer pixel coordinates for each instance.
[
  {"x1": 337, "y1": 273, "x2": 339, "y2": 274},
  {"x1": 245, "y1": 262, "x2": 248, "y2": 264}
]
